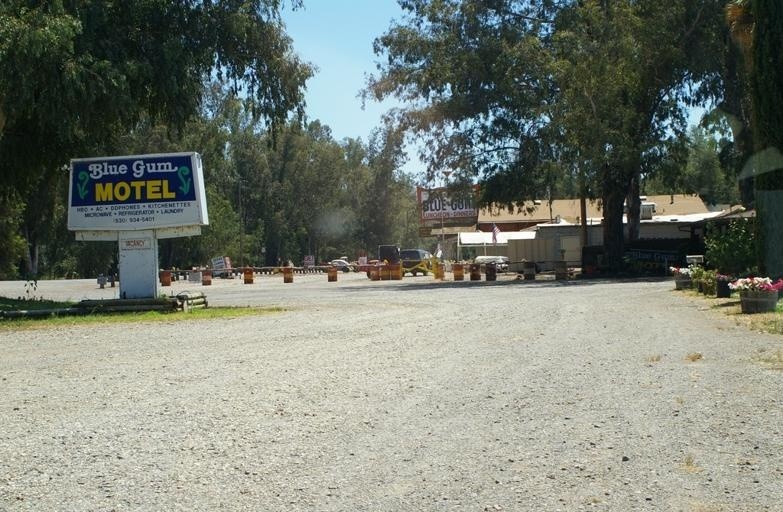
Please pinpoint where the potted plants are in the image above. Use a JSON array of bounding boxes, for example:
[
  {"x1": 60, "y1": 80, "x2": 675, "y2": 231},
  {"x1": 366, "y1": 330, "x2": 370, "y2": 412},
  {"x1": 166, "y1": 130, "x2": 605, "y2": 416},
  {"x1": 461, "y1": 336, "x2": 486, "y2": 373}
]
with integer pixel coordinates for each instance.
[{"x1": 691, "y1": 219, "x2": 759, "y2": 297}]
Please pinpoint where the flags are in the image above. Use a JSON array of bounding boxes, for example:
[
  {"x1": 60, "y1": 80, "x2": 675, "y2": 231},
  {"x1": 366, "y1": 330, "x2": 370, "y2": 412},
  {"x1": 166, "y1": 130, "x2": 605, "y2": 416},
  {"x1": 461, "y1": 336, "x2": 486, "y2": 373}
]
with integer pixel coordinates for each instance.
[{"x1": 492, "y1": 223, "x2": 500, "y2": 242}]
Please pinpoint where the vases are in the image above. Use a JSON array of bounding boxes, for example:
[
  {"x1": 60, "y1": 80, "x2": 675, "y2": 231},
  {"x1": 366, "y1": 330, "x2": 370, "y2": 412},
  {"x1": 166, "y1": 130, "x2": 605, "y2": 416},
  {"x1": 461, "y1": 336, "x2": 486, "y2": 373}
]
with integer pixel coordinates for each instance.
[
  {"x1": 675, "y1": 279, "x2": 692, "y2": 290},
  {"x1": 739, "y1": 291, "x2": 778, "y2": 314}
]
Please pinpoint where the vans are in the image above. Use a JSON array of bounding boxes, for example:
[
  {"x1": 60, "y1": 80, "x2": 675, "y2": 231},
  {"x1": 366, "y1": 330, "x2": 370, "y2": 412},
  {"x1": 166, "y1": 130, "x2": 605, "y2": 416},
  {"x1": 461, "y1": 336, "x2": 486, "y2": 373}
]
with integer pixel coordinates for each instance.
[
  {"x1": 331, "y1": 245, "x2": 435, "y2": 276},
  {"x1": 475, "y1": 255, "x2": 510, "y2": 273}
]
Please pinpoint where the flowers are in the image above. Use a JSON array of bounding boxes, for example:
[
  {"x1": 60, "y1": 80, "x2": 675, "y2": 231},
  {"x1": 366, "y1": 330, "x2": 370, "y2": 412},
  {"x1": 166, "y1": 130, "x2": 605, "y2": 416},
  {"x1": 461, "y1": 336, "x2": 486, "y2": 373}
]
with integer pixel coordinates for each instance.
[
  {"x1": 667, "y1": 264, "x2": 693, "y2": 281},
  {"x1": 726, "y1": 275, "x2": 783, "y2": 293}
]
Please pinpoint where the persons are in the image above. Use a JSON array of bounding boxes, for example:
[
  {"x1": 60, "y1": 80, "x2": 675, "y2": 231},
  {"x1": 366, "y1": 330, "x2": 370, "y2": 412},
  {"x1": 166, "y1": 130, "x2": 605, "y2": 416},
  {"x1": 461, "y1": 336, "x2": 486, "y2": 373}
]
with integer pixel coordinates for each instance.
[
  {"x1": 107, "y1": 265, "x2": 113, "y2": 281},
  {"x1": 160, "y1": 263, "x2": 200, "y2": 281},
  {"x1": 287, "y1": 260, "x2": 295, "y2": 267},
  {"x1": 277, "y1": 257, "x2": 283, "y2": 267},
  {"x1": 19, "y1": 257, "x2": 26, "y2": 279},
  {"x1": 111, "y1": 261, "x2": 117, "y2": 280}
]
